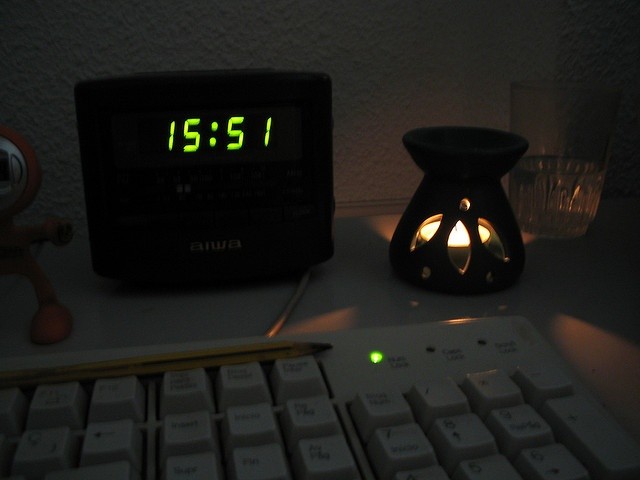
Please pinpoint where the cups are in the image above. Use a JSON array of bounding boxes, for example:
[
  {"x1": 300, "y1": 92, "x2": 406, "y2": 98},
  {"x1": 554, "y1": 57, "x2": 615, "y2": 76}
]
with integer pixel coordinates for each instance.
[{"x1": 507, "y1": 80, "x2": 621, "y2": 239}]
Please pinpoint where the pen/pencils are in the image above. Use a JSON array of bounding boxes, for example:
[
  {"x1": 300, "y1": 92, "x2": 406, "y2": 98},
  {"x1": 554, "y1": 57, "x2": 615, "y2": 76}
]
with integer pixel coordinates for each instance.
[{"x1": 0, "y1": 340, "x2": 334, "y2": 389}]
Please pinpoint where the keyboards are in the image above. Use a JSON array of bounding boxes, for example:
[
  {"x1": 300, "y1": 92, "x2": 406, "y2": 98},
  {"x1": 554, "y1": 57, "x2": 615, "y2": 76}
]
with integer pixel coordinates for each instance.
[{"x1": 0, "y1": 313, "x2": 640, "y2": 480}]
